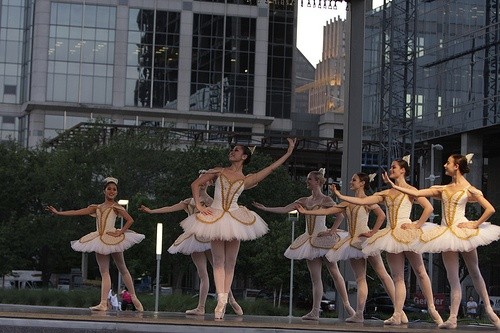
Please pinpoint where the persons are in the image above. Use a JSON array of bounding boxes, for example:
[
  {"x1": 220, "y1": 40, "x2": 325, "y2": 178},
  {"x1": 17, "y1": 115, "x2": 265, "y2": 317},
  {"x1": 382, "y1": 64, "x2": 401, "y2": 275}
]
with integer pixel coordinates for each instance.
[
  {"x1": 191, "y1": 136, "x2": 298, "y2": 317},
  {"x1": 252, "y1": 153, "x2": 500, "y2": 330},
  {"x1": 48, "y1": 178, "x2": 144, "y2": 312},
  {"x1": 138, "y1": 169, "x2": 243, "y2": 316}
]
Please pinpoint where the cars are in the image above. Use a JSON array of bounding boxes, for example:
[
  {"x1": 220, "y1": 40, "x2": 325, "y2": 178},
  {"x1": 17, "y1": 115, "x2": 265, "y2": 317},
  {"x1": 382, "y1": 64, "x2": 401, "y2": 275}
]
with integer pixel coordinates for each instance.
[{"x1": 281, "y1": 288, "x2": 428, "y2": 317}]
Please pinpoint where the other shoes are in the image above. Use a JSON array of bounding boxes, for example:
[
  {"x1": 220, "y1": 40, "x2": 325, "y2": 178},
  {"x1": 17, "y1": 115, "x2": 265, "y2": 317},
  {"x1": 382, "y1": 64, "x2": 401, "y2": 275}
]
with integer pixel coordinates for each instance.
[
  {"x1": 438, "y1": 320, "x2": 457, "y2": 328},
  {"x1": 401, "y1": 310, "x2": 408, "y2": 323},
  {"x1": 186, "y1": 309, "x2": 204, "y2": 315},
  {"x1": 428, "y1": 307, "x2": 443, "y2": 325},
  {"x1": 384, "y1": 317, "x2": 401, "y2": 324},
  {"x1": 485, "y1": 308, "x2": 500, "y2": 329},
  {"x1": 131, "y1": 296, "x2": 143, "y2": 312},
  {"x1": 89, "y1": 304, "x2": 107, "y2": 310},
  {"x1": 301, "y1": 313, "x2": 319, "y2": 319},
  {"x1": 345, "y1": 317, "x2": 363, "y2": 322},
  {"x1": 344, "y1": 305, "x2": 356, "y2": 316},
  {"x1": 215, "y1": 293, "x2": 228, "y2": 321},
  {"x1": 229, "y1": 299, "x2": 243, "y2": 315}
]
